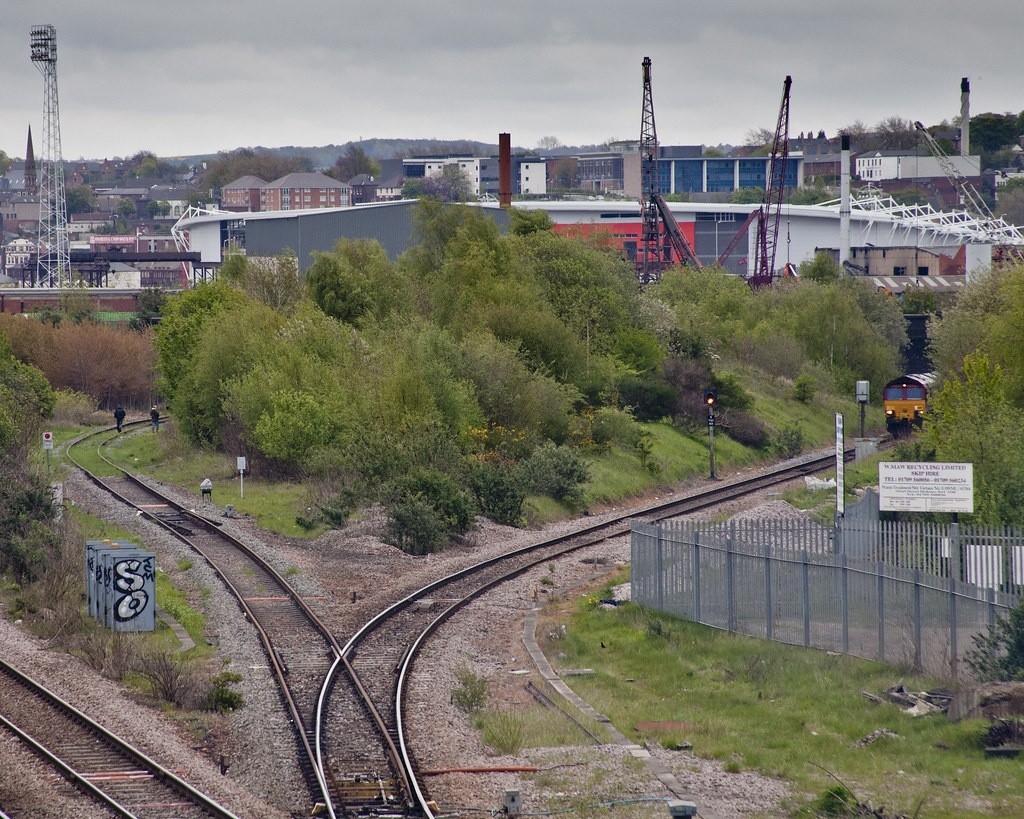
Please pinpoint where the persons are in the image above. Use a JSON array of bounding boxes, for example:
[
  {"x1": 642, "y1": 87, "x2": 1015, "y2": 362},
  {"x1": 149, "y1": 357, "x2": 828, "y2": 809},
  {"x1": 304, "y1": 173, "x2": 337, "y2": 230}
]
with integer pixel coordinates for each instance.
[
  {"x1": 150, "y1": 408, "x2": 160, "y2": 433},
  {"x1": 114, "y1": 406, "x2": 126, "y2": 433}
]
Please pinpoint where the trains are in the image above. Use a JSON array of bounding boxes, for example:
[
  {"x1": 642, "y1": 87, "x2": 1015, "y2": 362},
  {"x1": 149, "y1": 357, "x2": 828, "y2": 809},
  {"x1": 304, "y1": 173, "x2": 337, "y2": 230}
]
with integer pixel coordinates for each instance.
[{"x1": 883, "y1": 370, "x2": 942, "y2": 440}]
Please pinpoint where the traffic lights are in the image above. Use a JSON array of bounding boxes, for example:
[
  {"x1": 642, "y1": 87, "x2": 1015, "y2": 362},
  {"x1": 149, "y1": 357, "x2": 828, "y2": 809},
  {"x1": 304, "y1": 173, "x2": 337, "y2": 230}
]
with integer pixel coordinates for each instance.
[{"x1": 702, "y1": 386, "x2": 717, "y2": 405}]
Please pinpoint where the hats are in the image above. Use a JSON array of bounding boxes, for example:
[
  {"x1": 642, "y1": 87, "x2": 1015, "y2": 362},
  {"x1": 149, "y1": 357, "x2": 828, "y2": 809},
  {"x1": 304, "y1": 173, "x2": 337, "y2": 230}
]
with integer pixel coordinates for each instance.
[{"x1": 117, "y1": 406, "x2": 123, "y2": 409}]
